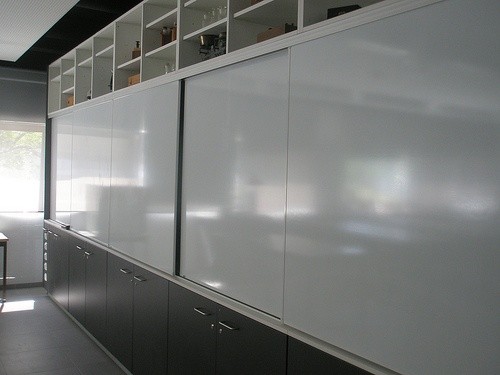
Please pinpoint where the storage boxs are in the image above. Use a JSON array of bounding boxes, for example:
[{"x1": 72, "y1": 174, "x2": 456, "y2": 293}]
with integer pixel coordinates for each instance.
[
  {"x1": 67, "y1": 96, "x2": 74, "y2": 106},
  {"x1": 257, "y1": 23, "x2": 297, "y2": 43},
  {"x1": 127, "y1": 74, "x2": 140, "y2": 86}
]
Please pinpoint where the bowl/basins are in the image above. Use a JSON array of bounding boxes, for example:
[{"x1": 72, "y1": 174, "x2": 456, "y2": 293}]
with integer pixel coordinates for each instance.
[{"x1": 200, "y1": 35, "x2": 217, "y2": 46}]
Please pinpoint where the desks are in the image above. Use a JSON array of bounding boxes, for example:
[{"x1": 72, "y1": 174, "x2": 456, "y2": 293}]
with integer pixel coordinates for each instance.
[{"x1": 0, "y1": 233, "x2": 9, "y2": 302}]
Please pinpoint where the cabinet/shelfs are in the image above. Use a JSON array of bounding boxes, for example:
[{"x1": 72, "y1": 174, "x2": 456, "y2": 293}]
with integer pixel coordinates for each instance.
[{"x1": 42, "y1": 0, "x2": 499, "y2": 375}]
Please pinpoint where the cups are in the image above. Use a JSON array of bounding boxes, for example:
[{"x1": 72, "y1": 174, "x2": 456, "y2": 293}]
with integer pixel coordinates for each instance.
[
  {"x1": 165, "y1": 63, "x2": 174, "y2": 74},
  {"x1": 203, "y1": 7, "x2": 227, "y2": 26}
]
